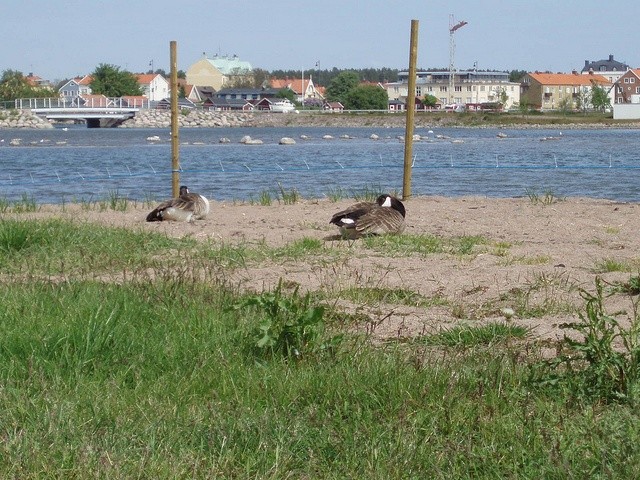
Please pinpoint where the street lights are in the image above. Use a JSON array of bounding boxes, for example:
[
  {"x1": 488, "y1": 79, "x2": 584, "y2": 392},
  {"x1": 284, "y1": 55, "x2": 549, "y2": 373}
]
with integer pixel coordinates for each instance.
[{"x1": 315, "y1": 60, "x2": 320, "y2": 106}]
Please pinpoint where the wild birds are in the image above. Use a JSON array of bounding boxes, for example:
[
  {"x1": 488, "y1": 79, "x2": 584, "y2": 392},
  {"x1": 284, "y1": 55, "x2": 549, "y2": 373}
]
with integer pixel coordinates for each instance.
[
  {"x1": 329, "y1": 194, "x2": 406, "y2": 239},
  {"x1": 146, "y1": 186, "x2": 209, "y2": 222}
]
